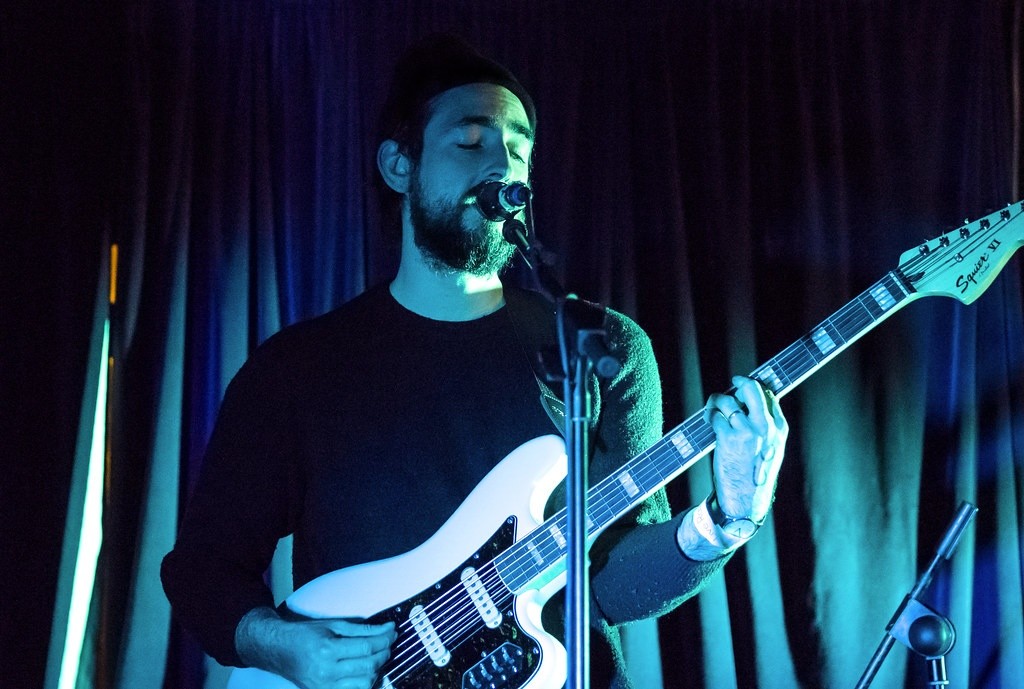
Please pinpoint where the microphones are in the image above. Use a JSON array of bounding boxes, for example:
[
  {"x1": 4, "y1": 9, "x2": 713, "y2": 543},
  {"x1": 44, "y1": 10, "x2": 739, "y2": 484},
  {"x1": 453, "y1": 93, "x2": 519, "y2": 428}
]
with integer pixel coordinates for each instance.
[{"x1": 475, "y1": 181, "x2": 533, "y2": 222}]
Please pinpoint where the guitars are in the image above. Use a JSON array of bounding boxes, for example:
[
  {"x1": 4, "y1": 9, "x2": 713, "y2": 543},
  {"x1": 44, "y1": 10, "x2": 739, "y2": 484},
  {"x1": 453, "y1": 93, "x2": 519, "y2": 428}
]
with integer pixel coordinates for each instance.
[{"x1": 218, "y1": 193, "x2": 1024, "y2": 689}]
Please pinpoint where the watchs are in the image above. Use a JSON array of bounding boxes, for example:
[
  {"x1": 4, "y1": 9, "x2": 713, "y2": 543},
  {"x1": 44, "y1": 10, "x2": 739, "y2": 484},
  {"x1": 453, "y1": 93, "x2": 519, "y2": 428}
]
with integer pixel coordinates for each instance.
[{"x1": 705, "y1": 489, "x2": 767, "y2": 542}]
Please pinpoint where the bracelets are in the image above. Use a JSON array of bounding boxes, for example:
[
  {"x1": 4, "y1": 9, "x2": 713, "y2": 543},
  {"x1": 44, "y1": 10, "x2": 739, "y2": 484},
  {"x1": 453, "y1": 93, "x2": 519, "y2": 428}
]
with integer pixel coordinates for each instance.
[{"x1": 695, "y1": 496, "x2": 759, "y2": 551}]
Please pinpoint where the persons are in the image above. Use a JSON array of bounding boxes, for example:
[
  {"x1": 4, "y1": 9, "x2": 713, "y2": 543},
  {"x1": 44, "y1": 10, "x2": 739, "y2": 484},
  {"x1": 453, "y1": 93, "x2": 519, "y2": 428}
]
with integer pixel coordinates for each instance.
[{"x1": 160, "y1": 47, "x2": 792, "y2": 689}]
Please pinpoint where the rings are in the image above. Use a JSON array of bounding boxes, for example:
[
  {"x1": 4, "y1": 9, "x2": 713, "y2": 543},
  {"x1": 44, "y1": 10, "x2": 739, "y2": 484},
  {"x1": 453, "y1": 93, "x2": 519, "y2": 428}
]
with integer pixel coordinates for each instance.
[{"x1": 727, "y1": 410, "x2": 746, "y2": 427}]
